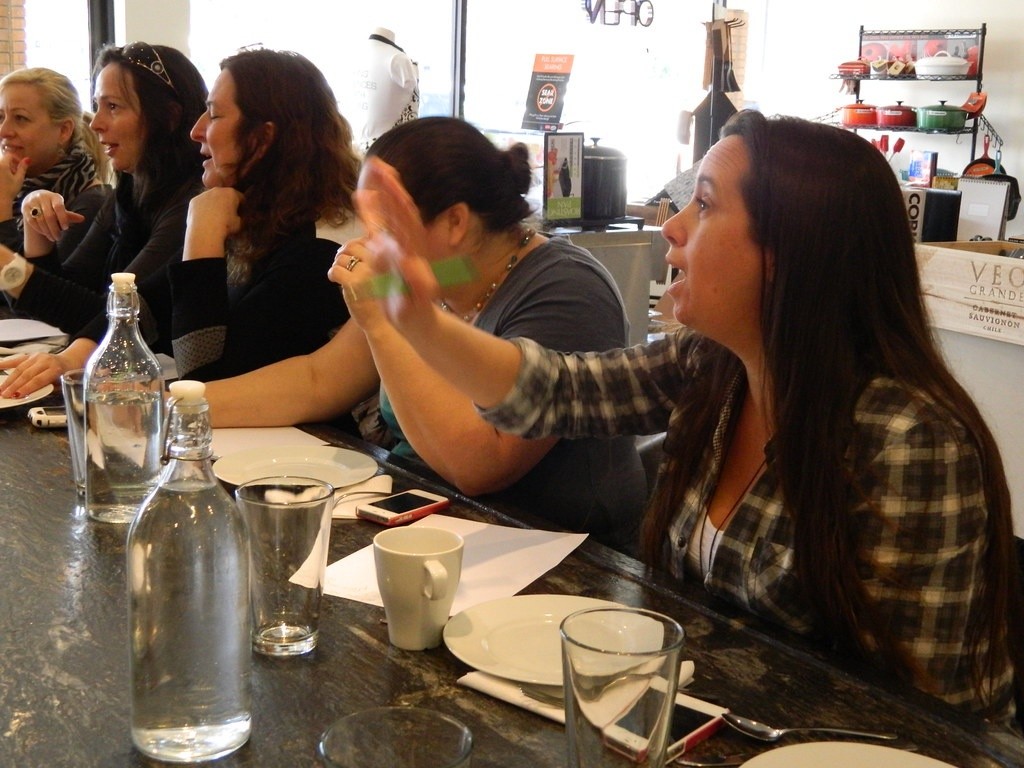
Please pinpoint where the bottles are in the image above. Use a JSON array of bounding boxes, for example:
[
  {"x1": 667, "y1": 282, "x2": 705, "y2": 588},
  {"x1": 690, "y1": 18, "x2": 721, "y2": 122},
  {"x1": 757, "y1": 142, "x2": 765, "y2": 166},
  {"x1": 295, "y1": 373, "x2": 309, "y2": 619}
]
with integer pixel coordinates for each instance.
[
  {"x1": 81, "y1": 272, "x2": 165, "y2": 525},
  {"x1": 126, "y1": 377, "x2": 251, "y2": 762}
]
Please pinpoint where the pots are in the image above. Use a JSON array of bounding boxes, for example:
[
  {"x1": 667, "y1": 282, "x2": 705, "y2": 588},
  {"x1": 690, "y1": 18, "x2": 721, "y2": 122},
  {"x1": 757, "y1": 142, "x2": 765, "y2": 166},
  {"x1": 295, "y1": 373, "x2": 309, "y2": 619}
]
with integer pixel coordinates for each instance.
[
  {"x1": 978, "y1": 150, "x2": 1021, "y2": 223},
  {"x1": 842, "y1": 99, "x2": 876, "y2": 125},
  {"x1": 961, "y1": 135, "x2": 1007, "y2": 178},
  {"x1": 582, "y1": 136, "x2": 627, "y2": 219},
  {"x1": 875, "y1": 100, "x2": 916, "y2": 126},
  {"x1": 915, "y1": 51, "x2": 972, "y2": 80},
  {"x1": 912, "y1": 100, "x2": 966, "y2": 129},
  {"x1": 837, "y1": 57, "x2": 870, "y2": 78}
]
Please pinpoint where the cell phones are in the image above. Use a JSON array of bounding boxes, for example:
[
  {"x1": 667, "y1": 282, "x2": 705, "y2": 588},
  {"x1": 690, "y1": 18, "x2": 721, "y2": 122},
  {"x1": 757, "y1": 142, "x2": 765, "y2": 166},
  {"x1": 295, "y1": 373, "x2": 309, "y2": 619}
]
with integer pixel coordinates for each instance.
[
  {"x1": 603, "y1": 676, "x2": 667, "y2": 763},
  {"x1": 356, "y1": 490, "x2": 449, "y2": 526},
  {"x1": 27, "y1": 405, "x2": 68, "y2": 427},
  {"x1": 665, "y1": 698, "x2": 731, "y2": 763}
]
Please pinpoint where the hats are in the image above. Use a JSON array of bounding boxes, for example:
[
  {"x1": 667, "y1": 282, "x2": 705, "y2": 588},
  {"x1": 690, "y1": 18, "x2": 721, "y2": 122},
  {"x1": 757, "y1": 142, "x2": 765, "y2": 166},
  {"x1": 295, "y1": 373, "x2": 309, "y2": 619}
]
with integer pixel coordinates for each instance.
[{"x1": 369, "y1": 27, "x2": 408, "y2": 55}]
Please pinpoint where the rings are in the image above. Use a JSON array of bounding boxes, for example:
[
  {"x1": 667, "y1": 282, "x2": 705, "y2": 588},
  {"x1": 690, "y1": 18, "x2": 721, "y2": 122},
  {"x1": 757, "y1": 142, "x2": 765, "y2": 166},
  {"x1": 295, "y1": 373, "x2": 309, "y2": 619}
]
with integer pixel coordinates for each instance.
[
  {"x1": 346, "y1": 256, "x2": 359, "y2": 271},
  {"x1": 30, "y1": 207, "x2": 42, "y2": 218}
]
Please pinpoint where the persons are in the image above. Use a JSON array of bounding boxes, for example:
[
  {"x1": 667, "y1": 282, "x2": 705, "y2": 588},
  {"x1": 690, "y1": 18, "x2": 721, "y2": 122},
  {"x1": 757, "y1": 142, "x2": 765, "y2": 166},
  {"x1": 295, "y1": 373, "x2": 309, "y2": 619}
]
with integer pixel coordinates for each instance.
[
  {"x1": 91, "y1": 116, "x2": 647, "y2": 553},
  {"x1": 0, "y1": 53, "x2": 361, "y2": 402},
  {"x1": 0, "y1": 41, "x2": 208, "y2": 333},
  {"x1": 355, "y1": 108, "x2": 1016, "y2": 732},
  {"x1": 0, "y1": 69, "x2": 113, "y2": 308}
]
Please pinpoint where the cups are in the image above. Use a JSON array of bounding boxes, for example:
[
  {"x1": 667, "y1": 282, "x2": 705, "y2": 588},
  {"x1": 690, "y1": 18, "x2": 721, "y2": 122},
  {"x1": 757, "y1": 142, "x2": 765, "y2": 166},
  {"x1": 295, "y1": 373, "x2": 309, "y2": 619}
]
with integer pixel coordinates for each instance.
[
  {"x1": 62, "y1": 368, "x2": 87, "y2": 497},
  {"x1": 318, "y1": 705, "x2": 473, "y2": 768},
  {"x1": 235, "y1": 475, "x2": 335, "y2": 654},
  {"x1": 558, "y1": 609, "x2": 684, "y2": 767},
  {"x1": 373, "y1": 526, "x2": 463, "y2": 649}
]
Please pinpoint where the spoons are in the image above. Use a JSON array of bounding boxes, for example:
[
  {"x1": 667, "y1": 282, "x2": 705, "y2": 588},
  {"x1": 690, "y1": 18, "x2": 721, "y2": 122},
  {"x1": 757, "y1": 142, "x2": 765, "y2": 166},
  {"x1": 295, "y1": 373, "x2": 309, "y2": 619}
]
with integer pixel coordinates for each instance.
[{"x1": 721, "y1": 712, "x2": 898, "y2": 741}]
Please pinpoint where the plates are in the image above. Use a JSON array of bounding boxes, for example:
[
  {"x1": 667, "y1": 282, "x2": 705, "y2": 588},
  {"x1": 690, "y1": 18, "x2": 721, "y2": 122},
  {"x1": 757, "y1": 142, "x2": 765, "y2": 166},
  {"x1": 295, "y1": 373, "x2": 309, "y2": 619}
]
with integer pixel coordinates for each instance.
[
  {"x1": 213, "y1": 445, "x2": 379, "y2": 487},
  {"x1": 441, "y1": 593, "x2": 665, "y2": 686},
  {"x1": 3, "y1": 344, "x2": 66, "y2": 375},
  {"x1": 738, "y1": 742, "x2": 960, "y2": 768},
  {"x1": 0, "y1": 375, "x2": 53, "y2": 408}
]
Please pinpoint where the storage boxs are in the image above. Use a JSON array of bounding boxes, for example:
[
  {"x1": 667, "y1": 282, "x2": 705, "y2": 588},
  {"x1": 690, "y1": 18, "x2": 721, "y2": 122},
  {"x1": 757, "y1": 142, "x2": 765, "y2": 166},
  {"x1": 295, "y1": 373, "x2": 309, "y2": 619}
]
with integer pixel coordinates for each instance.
[
  {"x1": 908, "y1": 149, "x2": 936, "y2": 189},
  {"x1": 912, "y1": 240, "x2": 1024, "y2": 345}
]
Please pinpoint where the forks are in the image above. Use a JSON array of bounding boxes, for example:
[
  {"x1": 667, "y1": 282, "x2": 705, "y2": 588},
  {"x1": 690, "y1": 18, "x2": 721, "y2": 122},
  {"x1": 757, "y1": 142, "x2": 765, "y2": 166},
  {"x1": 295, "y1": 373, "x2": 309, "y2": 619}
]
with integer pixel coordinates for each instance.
[{"x1": 520, "y1": 683, "x2": 566, "y2": 709}]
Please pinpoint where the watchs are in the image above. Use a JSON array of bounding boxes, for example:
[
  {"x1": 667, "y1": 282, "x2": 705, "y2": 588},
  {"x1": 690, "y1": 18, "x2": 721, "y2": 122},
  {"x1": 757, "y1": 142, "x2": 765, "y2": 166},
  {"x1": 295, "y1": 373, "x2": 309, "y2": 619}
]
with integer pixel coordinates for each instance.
[{"x1": 0, "y1": 252, "x2": 26, "y2": 291}]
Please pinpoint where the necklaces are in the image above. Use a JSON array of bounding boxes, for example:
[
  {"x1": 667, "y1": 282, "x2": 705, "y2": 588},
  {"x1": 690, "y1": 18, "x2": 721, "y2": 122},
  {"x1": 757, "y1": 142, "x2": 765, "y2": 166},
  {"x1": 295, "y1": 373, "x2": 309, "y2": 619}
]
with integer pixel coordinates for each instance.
[
  {"x1": 437, "y1": 226, "x2": 535, "y2": 322},
  {"x1": 699, "y1": 411, "x2": 764, "y2": 588}
]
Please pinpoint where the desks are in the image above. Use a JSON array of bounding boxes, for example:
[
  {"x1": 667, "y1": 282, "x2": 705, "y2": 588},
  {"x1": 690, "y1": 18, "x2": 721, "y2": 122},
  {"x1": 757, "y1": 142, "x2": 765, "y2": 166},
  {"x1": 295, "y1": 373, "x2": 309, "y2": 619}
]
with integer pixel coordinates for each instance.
[{"x1": 0, "y1": 309, "x2": 1024, "y2": 768}]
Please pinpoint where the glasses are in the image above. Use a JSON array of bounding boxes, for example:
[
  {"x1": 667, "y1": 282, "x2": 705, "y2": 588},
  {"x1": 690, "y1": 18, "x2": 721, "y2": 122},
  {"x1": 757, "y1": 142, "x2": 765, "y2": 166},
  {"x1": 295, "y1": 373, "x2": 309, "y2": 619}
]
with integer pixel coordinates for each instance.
[{"x1": 122, "y1": 39, "x2": 176, "y2": 88}]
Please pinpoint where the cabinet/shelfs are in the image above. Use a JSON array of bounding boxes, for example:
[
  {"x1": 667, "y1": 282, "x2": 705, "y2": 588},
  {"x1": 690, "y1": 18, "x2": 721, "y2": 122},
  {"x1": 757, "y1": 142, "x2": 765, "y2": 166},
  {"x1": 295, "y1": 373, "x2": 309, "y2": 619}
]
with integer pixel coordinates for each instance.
[{"x1": 840, "y1": 22, "x2": 989, "y2": 188}]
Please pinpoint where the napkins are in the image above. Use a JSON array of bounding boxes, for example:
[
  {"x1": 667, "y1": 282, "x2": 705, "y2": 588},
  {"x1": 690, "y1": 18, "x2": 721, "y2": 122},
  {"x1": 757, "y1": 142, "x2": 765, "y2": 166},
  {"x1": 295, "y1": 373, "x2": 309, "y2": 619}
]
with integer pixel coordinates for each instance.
[
  {"x1": 316, "y1": 513, "x2": 591, "y2": 616},
  {"x1": 112, "y1": 420, "x2": 331, "y2": 470},
  {"x1": 0, "y1": 318, "x2": 66, "y2": 343}
]
